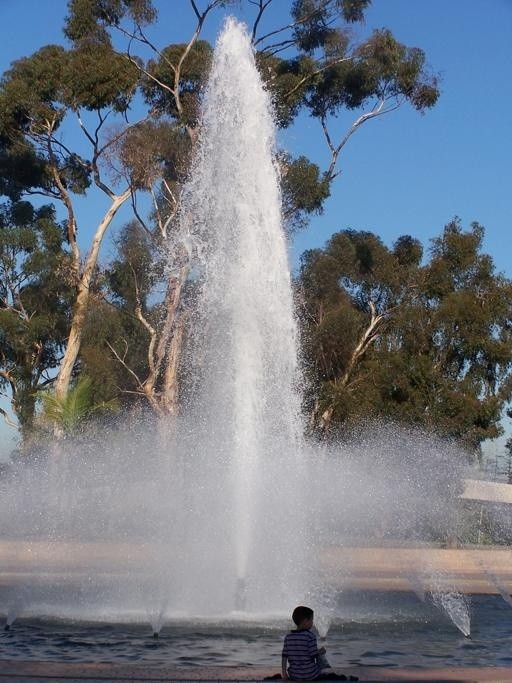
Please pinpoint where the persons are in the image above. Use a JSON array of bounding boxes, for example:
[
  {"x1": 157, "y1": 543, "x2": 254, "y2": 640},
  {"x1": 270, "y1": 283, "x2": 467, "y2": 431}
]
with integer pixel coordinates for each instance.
[{"x1": 281, "y1": 606, "x2": 326, "y2": 683}]
